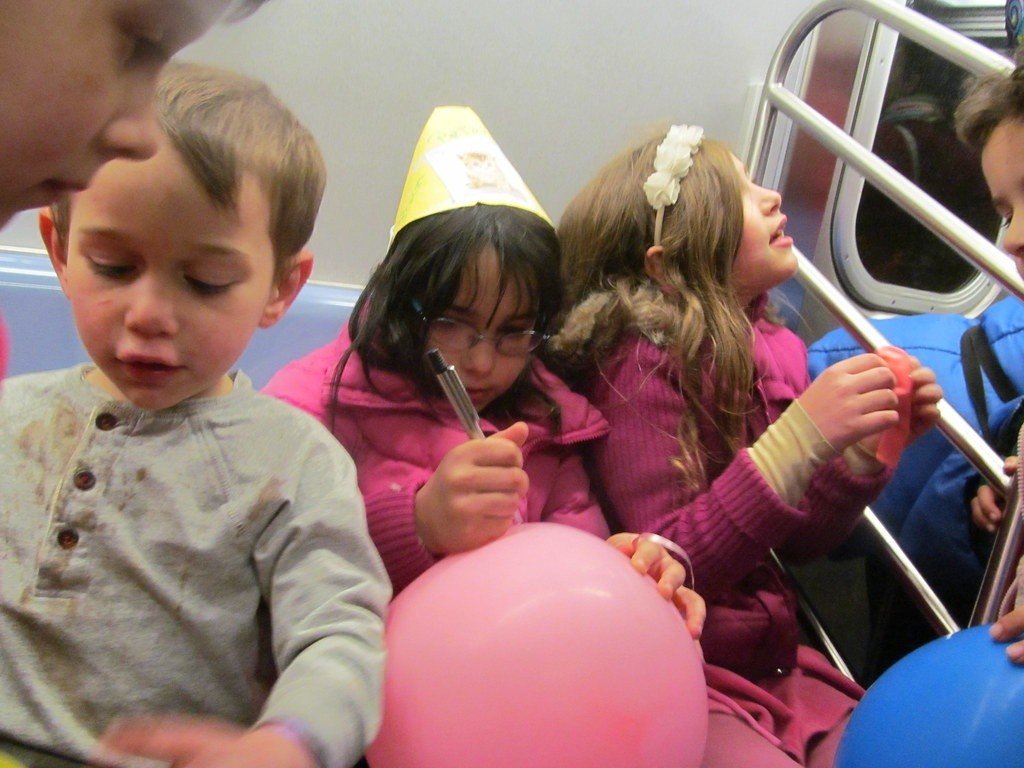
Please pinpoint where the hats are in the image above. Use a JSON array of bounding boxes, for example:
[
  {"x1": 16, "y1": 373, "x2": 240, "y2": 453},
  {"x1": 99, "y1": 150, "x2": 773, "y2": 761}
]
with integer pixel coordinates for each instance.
[{"x1": 386, "y1": 105, "x2": 557, "y2": 253}]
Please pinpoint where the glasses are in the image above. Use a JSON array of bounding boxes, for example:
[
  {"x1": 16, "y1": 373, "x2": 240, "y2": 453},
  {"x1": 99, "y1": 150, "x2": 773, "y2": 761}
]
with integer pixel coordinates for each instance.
[{"x1": 411, "y1": 298, "x2": 550, "y2": 356}]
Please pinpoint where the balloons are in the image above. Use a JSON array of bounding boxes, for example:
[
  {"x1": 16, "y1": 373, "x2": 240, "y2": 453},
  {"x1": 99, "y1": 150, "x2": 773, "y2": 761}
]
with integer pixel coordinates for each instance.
[
  {"x1": 364, "y1": 521, "x2": 708, "y2": 768},
  {"x1": 872, "y1": 346, "x2": 914, "y2": 470},
  {"x1": 833, "y1": 624, "x2": 1024, "y2": 768}
]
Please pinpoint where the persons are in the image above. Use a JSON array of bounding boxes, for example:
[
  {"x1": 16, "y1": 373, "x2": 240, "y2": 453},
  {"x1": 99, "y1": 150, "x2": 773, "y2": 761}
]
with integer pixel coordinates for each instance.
[
  {"x1": 0, "y1": 61, "x2": 392, "y2": 768},
  {"x1": 542, "y1": 127, "x2": 943, "y2": 768},
  {"x1": 0, "y1": 0, "x2": 262, "y2": 384},
  {"x1": 261, "y1": 107, "x2": 704, "y2": 649},
  {"x1": 953, "y1": 60, "x2": 1024, "y2": 667}
]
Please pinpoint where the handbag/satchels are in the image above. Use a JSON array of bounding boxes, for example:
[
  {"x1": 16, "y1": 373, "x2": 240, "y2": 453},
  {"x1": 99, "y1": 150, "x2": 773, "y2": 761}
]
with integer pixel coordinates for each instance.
[{"x1": 807, "y1": 295, "x2": 1024, "y2": 573}]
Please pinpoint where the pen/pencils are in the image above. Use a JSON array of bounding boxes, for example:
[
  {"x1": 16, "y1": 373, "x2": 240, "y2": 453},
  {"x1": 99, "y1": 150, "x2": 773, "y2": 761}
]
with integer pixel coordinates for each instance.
[{"x1": 423, "y1": 348, "x2": 525, "y2": 527}]
